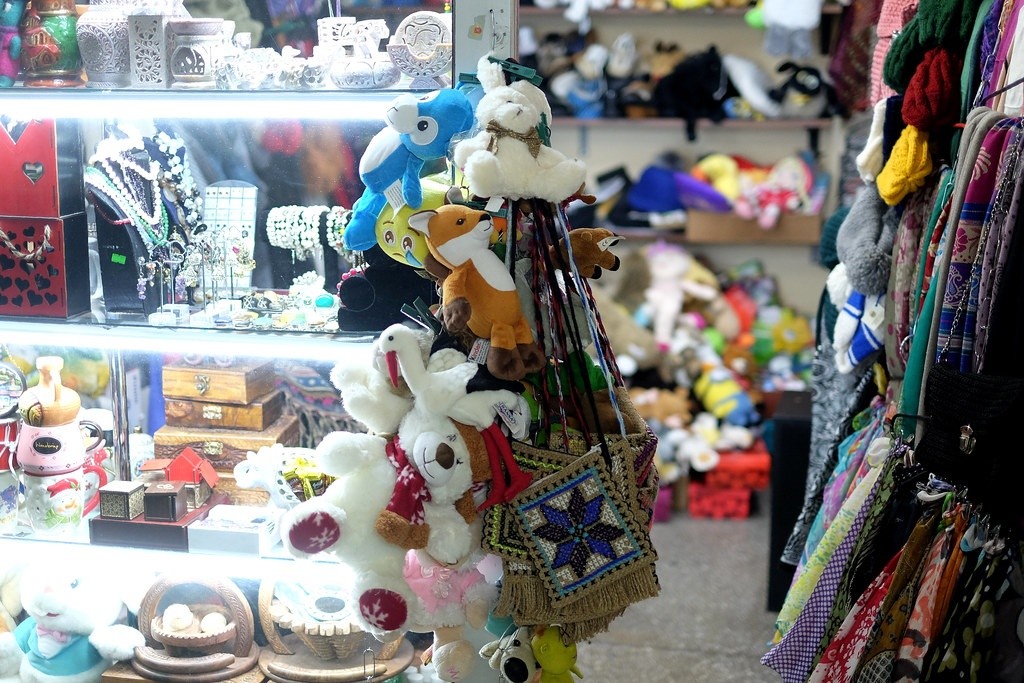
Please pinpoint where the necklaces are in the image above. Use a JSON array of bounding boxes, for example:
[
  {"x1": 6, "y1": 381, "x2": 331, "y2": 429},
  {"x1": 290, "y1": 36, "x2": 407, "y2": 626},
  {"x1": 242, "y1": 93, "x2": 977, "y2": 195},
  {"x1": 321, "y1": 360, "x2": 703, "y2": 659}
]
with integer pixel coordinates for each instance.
[{"x1": 83, "y1": 119, "x2": 204, "y2": 256}]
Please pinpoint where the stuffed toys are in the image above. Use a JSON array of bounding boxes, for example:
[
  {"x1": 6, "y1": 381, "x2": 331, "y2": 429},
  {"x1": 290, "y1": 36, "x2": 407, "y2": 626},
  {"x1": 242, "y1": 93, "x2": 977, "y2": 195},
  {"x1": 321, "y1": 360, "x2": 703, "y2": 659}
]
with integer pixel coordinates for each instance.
[
  {"x1": 234, "y1": 322, "x2": 587, "y2": 683},
  {"x1": 1, "y1": 534, "x2": 146, "y2": 683},
  {"x1": 342, "y1": 0, "x2": 864, "y2": 323},
  {"x1": 608, "y1": 323, "x2": 821, "y2": 484}
]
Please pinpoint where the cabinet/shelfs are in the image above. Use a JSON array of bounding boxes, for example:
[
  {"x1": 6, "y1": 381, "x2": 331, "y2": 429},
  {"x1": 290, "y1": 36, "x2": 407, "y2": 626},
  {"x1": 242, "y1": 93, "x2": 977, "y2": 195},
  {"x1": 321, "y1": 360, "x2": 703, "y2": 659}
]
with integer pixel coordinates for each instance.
[
  {"x1": 515, "y1": 0, "x2": 847, "y2": 245},
  {"x1": 0, "y1": 0, "x2": 457, "y2": 566}
]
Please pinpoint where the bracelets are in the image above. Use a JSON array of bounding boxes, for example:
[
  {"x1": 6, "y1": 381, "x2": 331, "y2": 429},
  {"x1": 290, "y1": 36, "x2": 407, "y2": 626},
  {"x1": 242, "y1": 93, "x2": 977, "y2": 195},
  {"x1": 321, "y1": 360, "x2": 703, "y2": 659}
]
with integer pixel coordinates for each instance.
[{"x1": 266, "y1": 201, "x2": 365, "y2": 271}]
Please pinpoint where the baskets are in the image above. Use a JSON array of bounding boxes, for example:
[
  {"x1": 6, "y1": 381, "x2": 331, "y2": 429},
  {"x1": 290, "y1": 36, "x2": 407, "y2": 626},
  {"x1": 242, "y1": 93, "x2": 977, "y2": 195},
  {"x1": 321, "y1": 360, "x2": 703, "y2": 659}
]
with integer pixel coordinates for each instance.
[{"x1": 284, "y1": 396, "x2": 369, "y2": 453}]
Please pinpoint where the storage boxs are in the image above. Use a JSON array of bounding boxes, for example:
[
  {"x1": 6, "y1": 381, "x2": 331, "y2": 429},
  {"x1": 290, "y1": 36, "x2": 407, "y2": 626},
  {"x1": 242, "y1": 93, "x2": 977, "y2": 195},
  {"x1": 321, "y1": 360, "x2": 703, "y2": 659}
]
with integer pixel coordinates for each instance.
[
  {"x1": 88, "y1": 357, "x2": 301, "y2": 555},
  {"x1": 685, "y1": 210, "x2": 822, "y2": 245}
]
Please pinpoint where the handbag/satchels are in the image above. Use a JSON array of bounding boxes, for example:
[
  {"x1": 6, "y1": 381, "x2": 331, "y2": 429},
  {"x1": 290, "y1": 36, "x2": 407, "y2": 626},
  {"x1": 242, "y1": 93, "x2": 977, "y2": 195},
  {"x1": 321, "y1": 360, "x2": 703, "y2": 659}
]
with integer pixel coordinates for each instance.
[{"x1": 483, "y1": 381, "x2": 660, "y2": 647}]
